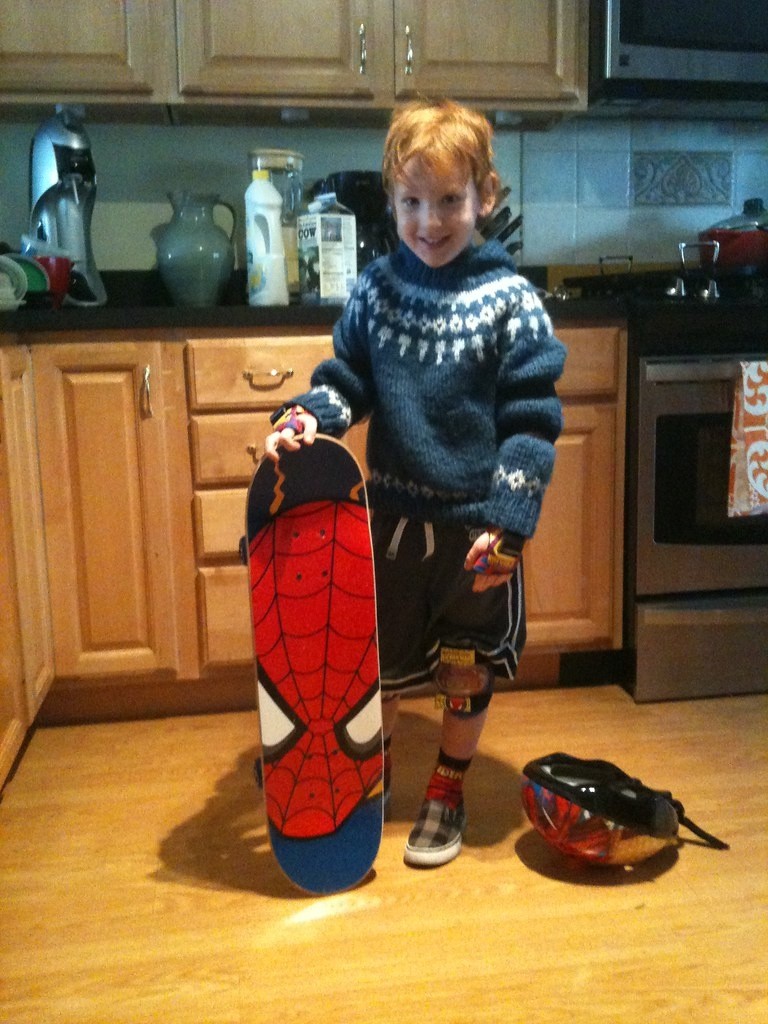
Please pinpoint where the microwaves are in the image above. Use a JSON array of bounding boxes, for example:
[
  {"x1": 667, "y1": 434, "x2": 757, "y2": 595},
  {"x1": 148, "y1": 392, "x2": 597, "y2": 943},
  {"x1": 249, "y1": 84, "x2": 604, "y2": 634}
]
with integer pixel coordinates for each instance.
[{"x1": 588, "y1": 0, "x2": 768, "y2": 102}]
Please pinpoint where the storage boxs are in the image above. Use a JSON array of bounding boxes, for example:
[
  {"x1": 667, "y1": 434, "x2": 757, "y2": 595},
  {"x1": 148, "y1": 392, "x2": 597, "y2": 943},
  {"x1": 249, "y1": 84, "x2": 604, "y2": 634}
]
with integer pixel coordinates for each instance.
[{"x1": 296, "y1": 191, "x2": 359, "y2": 306}]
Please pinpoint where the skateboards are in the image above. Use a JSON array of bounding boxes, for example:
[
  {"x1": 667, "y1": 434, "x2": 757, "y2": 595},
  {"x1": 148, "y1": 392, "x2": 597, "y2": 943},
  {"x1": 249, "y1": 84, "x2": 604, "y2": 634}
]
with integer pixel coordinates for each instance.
[{"x1": 238, "y1": 431, "x2": 383, "y2": 894}]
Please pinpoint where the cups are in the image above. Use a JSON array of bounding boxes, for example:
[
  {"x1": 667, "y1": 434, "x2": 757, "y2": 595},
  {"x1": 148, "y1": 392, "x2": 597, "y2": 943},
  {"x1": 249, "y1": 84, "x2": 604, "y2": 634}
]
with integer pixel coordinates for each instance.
[{"x1": 39, "y1": 256, "x2": 72, "y2": 309}]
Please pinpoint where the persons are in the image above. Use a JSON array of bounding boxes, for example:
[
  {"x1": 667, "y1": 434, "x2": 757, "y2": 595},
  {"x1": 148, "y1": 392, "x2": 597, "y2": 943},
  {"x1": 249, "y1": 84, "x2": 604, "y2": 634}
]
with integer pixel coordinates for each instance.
[{"x1": 264, "y1": 96, "x2": 567, "y2": 864}]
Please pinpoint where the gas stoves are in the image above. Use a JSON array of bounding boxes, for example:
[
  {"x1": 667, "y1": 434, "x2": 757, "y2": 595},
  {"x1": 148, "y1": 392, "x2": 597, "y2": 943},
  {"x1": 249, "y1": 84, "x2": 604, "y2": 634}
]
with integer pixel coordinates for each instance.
[{"x1": 542, "y1": 269, "x2": 768, "y2": 357}]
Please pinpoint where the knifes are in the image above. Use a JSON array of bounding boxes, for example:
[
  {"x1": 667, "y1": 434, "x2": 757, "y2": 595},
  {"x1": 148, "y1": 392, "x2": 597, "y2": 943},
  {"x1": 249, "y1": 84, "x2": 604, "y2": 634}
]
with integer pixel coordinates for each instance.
[{"x1": 475, "y1": 187, "x2": 524, "y2": 255}]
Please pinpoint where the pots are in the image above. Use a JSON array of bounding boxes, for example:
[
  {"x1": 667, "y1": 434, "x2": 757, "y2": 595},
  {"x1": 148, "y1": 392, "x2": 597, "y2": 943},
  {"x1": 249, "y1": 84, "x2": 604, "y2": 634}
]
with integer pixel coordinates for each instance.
[{"x1": 707, "y1": 199, "x2": 768, "y2": 268}]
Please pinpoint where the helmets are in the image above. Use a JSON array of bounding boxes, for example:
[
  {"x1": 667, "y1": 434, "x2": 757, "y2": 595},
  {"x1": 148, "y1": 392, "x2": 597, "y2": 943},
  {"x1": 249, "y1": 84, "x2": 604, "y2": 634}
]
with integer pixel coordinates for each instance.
[{"x1": 519, "y1": 743, "x2": 732, "y2": 877}]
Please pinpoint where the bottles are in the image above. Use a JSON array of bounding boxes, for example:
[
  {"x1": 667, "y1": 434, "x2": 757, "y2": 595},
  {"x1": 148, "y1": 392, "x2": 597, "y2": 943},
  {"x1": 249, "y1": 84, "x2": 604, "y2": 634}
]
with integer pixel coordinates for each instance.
[{"x1": 251, "y1": 150, "x2": 304, "y2": 300}]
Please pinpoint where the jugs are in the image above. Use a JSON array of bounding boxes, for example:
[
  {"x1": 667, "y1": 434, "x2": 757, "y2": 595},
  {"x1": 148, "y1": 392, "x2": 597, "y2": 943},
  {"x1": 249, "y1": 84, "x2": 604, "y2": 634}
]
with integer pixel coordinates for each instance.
[{"x1": 156, "y1": 190, "x2": 237, "y2": 307}]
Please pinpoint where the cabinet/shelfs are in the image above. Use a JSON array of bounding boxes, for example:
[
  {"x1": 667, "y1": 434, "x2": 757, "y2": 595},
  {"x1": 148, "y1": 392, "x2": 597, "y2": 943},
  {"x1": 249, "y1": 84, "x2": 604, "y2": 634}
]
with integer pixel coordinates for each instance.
[
  {"x1": 0, "y1": 268, "x2": 632, "y2": 795},
  {"x1": 0, "y1": 0, "x2": 590, "y2": 132}
]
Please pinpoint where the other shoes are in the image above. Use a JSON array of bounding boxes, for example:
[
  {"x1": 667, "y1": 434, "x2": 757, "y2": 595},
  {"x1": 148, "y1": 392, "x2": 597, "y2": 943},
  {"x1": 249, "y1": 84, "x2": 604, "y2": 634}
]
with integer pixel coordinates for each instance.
[
  {"x1": 404, "y1": 797, "x2": 466, "y2": 866},
  {"x1": 383, "y1": 766, "x2": 392, "y2": 801}
]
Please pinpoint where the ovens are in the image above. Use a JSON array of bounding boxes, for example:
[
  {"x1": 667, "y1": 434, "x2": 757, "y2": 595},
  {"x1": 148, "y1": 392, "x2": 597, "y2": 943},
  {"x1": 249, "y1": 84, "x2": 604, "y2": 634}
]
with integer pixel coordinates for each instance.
[{"x1": 628, "y1": 358, "x2": 768, "y2": 702}]
